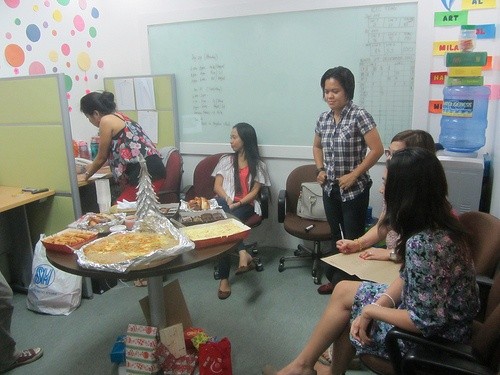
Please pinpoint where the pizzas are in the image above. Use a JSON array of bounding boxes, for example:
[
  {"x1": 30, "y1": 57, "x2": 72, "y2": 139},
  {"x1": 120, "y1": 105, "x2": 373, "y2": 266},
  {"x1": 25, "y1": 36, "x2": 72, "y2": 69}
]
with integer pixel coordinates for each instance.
[{"x1": 83, "y1": 232, "x2": 179, "y2": 264}]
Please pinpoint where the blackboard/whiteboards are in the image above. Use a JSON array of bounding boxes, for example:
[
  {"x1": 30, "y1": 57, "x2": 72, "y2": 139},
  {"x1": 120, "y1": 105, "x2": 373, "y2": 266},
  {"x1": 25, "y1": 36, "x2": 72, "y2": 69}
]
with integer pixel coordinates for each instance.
[{"x1": 140, "y1": 1, "x2": 435, "y2": 160}]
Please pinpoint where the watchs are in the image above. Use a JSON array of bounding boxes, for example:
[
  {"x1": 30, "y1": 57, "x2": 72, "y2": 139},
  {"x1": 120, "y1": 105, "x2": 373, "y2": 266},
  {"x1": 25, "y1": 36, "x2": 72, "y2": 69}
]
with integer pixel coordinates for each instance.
[
  {"x1": 389, "y1": 248, "x2": 398, "y2": 261},
  {"x1": 315, "y1": 167, "x2": 324, "y2": 175},
  {"x1": 84, "y1": 171, "x2": 91, "y2": 178}
]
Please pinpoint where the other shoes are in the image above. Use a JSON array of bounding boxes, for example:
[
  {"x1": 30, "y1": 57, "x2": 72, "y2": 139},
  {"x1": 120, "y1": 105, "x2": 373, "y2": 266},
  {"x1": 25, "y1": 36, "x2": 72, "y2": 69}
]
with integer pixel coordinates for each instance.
[
  {"x1": 218, "y1": 279, "x2": 232, "y2": 300},
  {"x1": 235, "y1": 261, "x2": 256, "y2": 275}
]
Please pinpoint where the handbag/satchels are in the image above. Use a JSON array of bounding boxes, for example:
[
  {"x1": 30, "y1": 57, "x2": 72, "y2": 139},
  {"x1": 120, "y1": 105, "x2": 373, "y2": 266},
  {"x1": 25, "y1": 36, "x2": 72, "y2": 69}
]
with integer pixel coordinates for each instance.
[
  {"x1": 25, "y1": 233, "x2": 82, "y2": 316},
  {"x1": 295, "y1": 181, "x2": 327, "y2": 222},
  {"x1": 197, "y1": 337, "x2": 233, "y2": 375}
]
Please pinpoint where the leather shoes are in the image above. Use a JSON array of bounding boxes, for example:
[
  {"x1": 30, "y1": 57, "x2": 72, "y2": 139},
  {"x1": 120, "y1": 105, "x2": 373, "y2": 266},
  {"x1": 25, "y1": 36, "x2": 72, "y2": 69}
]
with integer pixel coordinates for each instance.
[{"x1": 318, "y1": 283, "x2": 334, "y2": 294}]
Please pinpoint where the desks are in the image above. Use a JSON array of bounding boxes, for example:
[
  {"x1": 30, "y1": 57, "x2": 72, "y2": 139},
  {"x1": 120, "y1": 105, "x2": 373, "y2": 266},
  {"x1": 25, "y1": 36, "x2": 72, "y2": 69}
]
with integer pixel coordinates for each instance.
[
  {"x1": 0, "y1": 185, "x2": 55, "y2": 294},
  {"x1": 77, "y1": 165, "x2": 112, "y2": 187}
]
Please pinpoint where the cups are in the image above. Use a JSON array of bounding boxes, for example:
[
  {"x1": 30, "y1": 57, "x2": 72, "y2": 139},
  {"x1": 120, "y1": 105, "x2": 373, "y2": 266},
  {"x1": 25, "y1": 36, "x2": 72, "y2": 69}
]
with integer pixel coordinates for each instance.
[
  {"x1": 91, "y1": 139, "x2": 99, "y2": 160},
  {"x1": 79, "y1": 140, "x2": 90, "y2": 160}
]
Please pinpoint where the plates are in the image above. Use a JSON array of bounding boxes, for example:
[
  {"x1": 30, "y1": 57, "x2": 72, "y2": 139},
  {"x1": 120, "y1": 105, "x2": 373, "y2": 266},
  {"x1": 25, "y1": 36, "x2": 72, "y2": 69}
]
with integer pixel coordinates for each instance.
[{"x1": 108, "y1": 225, "x2": 127, "y2": 233}]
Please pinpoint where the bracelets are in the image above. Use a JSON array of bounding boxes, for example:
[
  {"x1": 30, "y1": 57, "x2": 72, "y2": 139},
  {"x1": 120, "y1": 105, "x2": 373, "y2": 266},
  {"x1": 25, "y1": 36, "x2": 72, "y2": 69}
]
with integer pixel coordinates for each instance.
[
  {"x1": 371, "y1": 293, "x2": 397, "y2": 309},
  {"x1": 238, "y1": 201, "x2": 243, "y2": 206},
  {"x1": 354, "y1": 239, "x2": 362, "y2": 251},
  {"x1": 226, "y1": 196, "x2": 231, "y2": 202}
]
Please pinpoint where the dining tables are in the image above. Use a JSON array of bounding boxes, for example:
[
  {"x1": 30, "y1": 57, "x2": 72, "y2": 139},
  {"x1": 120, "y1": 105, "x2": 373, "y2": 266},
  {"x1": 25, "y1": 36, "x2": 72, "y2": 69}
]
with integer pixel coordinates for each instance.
[{"x1": 47, "y1": 203, "x2": 244, "y2": 324}]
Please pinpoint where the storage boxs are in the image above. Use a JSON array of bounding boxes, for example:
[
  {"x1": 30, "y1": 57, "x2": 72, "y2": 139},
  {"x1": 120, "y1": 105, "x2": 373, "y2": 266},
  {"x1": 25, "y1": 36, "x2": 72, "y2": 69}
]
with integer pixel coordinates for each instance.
[
  {"x1": 125, "y1": 324, "x2": 158, "y2": 374},
  {"x1": 153, "y1": 344, "x2": 195, "y2": 375},
  {"x1": 111, "y1": 335, "x2": 126, "y2": 362},
  {"x1": 198, "y1": 340, "x2": 232, "y2": 374}
]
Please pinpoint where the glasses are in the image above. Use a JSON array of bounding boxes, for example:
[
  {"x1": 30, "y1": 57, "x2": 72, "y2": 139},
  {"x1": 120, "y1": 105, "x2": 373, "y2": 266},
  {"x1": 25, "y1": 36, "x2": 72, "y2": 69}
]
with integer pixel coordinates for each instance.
[{"x1": 384, "y1": 150, "x2": 396, "y2": 157}]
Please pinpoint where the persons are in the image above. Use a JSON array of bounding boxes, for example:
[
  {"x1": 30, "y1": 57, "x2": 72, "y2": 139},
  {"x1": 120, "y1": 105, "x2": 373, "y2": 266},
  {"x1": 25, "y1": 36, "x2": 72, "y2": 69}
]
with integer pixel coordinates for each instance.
[
  {"x1": 311, "y1": 66, "x2": 385, "y2": 295},
  {"x1": 208, "y1": 122, "x2": 273, "y2": 299},
  {"x1": 0, "y1": 271, "x2": 44, "y2": 375},
  {"x1": 317, "y1": 129, "x2": 461, "y2": 369},
  {"x1": 274, "y1": 145, "x2": 481, "y2": 375},
  {"x1": 75, "y1": 90, "x2": 169, "y2": 287}
]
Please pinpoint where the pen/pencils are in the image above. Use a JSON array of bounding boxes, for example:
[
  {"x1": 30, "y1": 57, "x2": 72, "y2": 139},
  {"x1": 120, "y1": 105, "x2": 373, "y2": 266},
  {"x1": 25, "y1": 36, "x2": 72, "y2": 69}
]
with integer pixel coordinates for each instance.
[{"x1": 338, "y1": 224, "x2": 350, "y2": 255}]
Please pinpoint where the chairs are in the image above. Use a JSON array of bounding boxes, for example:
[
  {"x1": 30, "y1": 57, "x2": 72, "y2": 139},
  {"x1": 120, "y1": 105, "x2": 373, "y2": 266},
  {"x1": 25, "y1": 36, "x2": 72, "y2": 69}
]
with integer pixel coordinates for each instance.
[
  {"x1": 385, "y1": 264, "x2": 500, "y2": 375},
  {"x1": 185, "y1": 152, "x2": 270, "y2": 280},
  {"x1": 456, "y1": 209, "x2": 500, "y2": 275},
  {"x1": 151, "y1": 148, "x2": 183, "y2": 202},
  {"x1": 278, "y1": 164, "x2": 343, "y2": 284}
]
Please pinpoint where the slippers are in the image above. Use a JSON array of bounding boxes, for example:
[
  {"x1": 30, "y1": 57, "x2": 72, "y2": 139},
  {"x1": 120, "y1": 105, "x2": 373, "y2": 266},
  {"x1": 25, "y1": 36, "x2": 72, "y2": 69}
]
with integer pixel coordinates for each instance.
[{"x1": 317, "y1": 347, "x2": 362, "y2": 368}]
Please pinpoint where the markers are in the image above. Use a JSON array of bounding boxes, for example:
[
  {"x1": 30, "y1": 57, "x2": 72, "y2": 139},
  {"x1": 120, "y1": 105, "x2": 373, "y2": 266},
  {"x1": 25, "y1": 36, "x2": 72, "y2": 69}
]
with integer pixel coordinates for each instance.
[{"x1": 304, "y1": 223, "x2": 316, "y2": 232}]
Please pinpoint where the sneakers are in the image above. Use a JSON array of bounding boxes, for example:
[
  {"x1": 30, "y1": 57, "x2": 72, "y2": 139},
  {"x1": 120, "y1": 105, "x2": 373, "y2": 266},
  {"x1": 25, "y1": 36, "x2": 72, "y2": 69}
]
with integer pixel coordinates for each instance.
[{"x1": 11, "y1": 346, "x2": 42, "y2": 367}]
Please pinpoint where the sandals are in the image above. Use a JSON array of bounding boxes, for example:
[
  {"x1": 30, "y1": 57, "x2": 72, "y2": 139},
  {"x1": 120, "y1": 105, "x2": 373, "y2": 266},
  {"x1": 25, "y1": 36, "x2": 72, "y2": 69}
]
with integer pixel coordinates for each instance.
[{"x1": 134, "y1": 278, "x2": 148, "y2": 286}]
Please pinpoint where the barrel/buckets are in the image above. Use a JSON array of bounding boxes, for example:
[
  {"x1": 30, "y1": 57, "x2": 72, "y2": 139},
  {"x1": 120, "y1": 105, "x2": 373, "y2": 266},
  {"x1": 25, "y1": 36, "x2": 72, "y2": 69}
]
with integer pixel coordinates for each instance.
[{"x1": 439, "y1": 85, "x2": 490, "y2": 155}]
